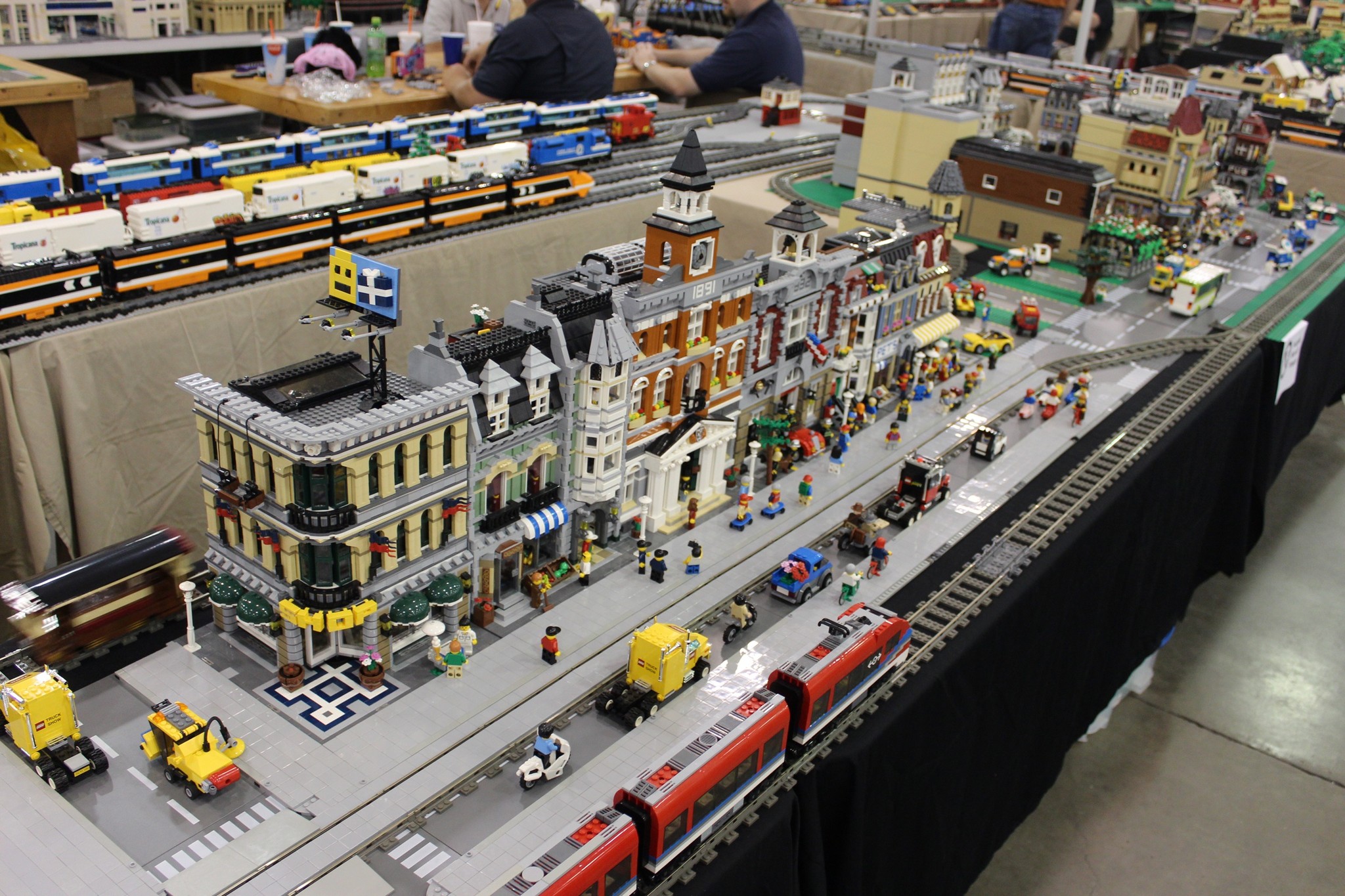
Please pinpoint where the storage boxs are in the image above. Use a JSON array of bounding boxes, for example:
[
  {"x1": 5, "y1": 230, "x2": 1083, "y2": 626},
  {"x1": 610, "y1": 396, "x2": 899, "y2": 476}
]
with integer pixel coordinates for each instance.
[
  {"x1": 166, "y1": 96, "x2": 261, "y2": 147},
  {"x1": 49, "y1": 66, "x2": 137, "y2": 139},
  {"x1": 114, "y1": 114, "x2": 184, "y2": 143},
  {"x1": 101, "y1": 134, "x2": 192, "y2": 162}
]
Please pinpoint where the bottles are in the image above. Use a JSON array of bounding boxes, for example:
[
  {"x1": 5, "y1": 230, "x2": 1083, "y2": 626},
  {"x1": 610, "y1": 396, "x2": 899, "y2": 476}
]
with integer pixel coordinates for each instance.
[{"x1": 365, "y1": 16, "x2": 385, "y2": 78}]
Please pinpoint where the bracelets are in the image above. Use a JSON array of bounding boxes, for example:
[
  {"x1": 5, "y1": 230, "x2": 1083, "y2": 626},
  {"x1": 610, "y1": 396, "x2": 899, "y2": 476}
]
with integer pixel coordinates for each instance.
[{"x1": 640, "y1": 59, "x2": 660, "y2": 71}]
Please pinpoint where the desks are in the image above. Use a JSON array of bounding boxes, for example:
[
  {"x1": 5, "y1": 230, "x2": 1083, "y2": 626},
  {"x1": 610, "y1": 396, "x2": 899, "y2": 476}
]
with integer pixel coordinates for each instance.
[
  {"x1": 192, "y1": 62, "x2": 676, "y2": 136},
  {"x1": 1, "y1": 54, "x2": 90, "y2": 192}
]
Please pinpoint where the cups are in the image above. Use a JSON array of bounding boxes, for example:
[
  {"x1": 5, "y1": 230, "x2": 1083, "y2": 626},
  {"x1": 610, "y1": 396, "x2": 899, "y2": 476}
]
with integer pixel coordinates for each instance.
[
  {"x1": 441, "y1": 30, "x2": 464, "y2": 68},
  {"x1": 399, "y1": 31, "x2": 423, "y2": 56},
  {"x1": 302, "y1": 23, "x2": 325, "y2": 55},
  {"x1": 328, "y1": 20, "x2": 354, "y2": 42},
  {"x1": 467, "y1": 19, "x2": 494, "y2": 51},
  {"x1": 261, "y1": 34, "x2": 289, "y2": 87}
]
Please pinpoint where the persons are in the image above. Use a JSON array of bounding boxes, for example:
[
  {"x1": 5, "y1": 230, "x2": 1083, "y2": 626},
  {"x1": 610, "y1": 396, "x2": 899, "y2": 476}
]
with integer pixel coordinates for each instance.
[
  {"x1": 629, "y1": 1, "x2": 806, "y2": 104},
  {"x1": 989, "y1": 1, "x2": 1080, "y2": 61},
  {"x1": 1057, "y1": 0, "x2": 1115, "y2": 65},
  {"x1": 1291, "y1": 0, "x2": 1319, "y2": 30},
  {"x1": 497, "y1": 174, "x2": 1334, "y2": 788},
  {"x1": 445, "y1": 1, "x2": 618, "y2": 104}
]
